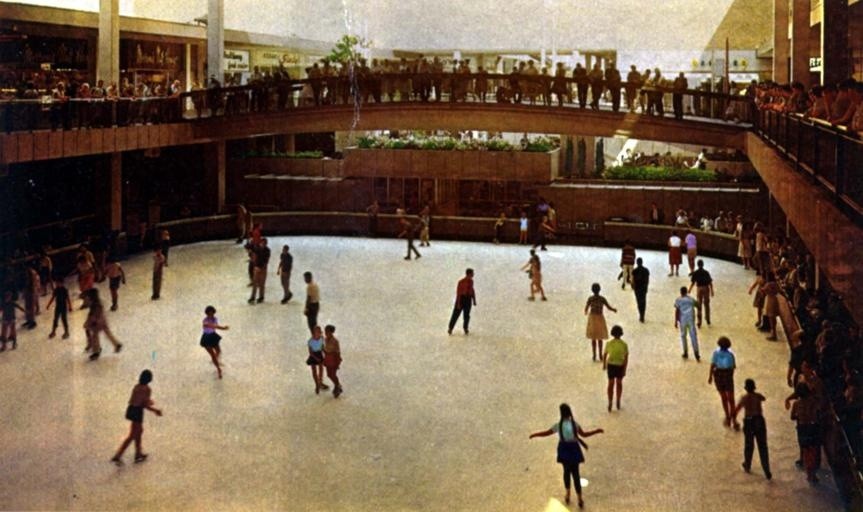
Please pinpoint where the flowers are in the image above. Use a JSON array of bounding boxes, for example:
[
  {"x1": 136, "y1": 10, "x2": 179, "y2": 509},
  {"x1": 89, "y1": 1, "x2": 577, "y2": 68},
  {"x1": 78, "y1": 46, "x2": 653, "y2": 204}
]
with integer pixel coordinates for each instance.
[{"x1": 355, "y1": 132, "x2": 559, "y2": 152}]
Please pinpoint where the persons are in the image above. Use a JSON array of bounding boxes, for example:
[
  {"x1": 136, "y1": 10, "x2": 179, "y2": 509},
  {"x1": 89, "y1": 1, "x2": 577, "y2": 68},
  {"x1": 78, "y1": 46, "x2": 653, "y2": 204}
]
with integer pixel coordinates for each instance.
[
  {"x1": 785, "y1": 292, "x2": 862, "y2": 487},
  {"x1": 747, "y1": 234, "x2": 814, "y2": 341},
  {"x1": 602, "y1": 325, "x2": 629, "y2": 413},
  {"x1": 1, "y1": 206, "x2": 194, "y2": 360},
  {"x1": 708, "y1": 336, "x2": 740, "y2": 431},
  {"x1": 618, "y1": 236, "x2": 635, "y2": 290},
  {"x1": 667, "y1": 229, "x2": 684, "y2": 276},
  {"x1": 650, "y1": 204, "x2": 666, "y2": 225},
  {"x1": 584, "y1": 282, "x2": 618, "y2": 363},
  {"x1": 366, "y1": 199, "x2": 379, "y2": 237},
  {"x1": 446, "y1": 267, "x2": 477, "y2": 335},
  {"x1": 674, "y1": 205, "x2": 768, "y2": 270},
  {"x1": 110, "y1": 369, "x2": 163, "y2": 465},
  {"x1": 721, "y1": 379, "x2": 772, "y2": 480},
  {"x1": 0, "y1": 52, "x2": 863, "y2": 183},
  {"x1": 305, "y1": 324, "x2": 330, "y2": 394},
  {"x1": 200, "y1": 305, "x2": 229, "y2": 379},
  {"x1": 395, "y1": 198, "x2": 436, "y2": 260},
  {"x1": 674, "y1": 286, "x2": 701, "y2": 363},
  {"x1": 688, "y1": 259, "x2": 714, "y2": 329},
  {"x1": 234, "y1": 200, "x2": 293, "y2": 304},
  {"x1": 630, "y1": 257, "x2": 650, "y2": 323},
  {"x1": 494, "y1": 197, "x2": 558, "y2": 301},
  {"x1": 302, "y1": 271, "x2": 320, "y2": 334},
  {"x1": 685, "y1": 230, "x2": 696, "y2": 275},
  {"x1": 323, "y1": 325, "x2": 344, "y2": 395},
  {"x1": 528, "y1": 402, "x2": 605, "y2": 510}
]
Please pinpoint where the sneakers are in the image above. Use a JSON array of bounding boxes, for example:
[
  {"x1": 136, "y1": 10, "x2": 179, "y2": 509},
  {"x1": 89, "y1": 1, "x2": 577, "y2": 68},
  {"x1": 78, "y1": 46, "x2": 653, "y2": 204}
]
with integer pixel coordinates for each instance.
[
  {"x1": 108, "y1": 457, "x2": 125, "y2": 469},
  {"x1": 133, "y1": 454, "x2": 147, "y2": 463}
]
